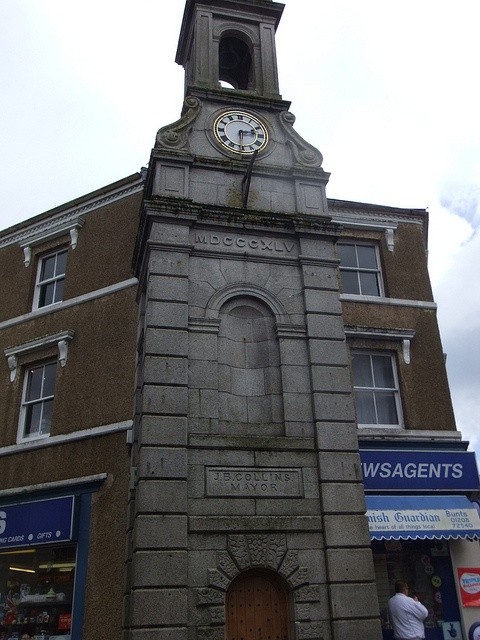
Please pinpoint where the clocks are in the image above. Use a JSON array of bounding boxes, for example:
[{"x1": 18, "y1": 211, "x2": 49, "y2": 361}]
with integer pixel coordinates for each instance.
[{"x1": 204, "y1": 105, "x2": 277, "y2": 162}]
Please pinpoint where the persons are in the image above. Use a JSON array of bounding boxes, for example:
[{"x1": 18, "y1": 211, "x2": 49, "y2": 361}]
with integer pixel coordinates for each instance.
[{"x1": 388, "y1": 580, "x2": 429, "y2": 640}]
